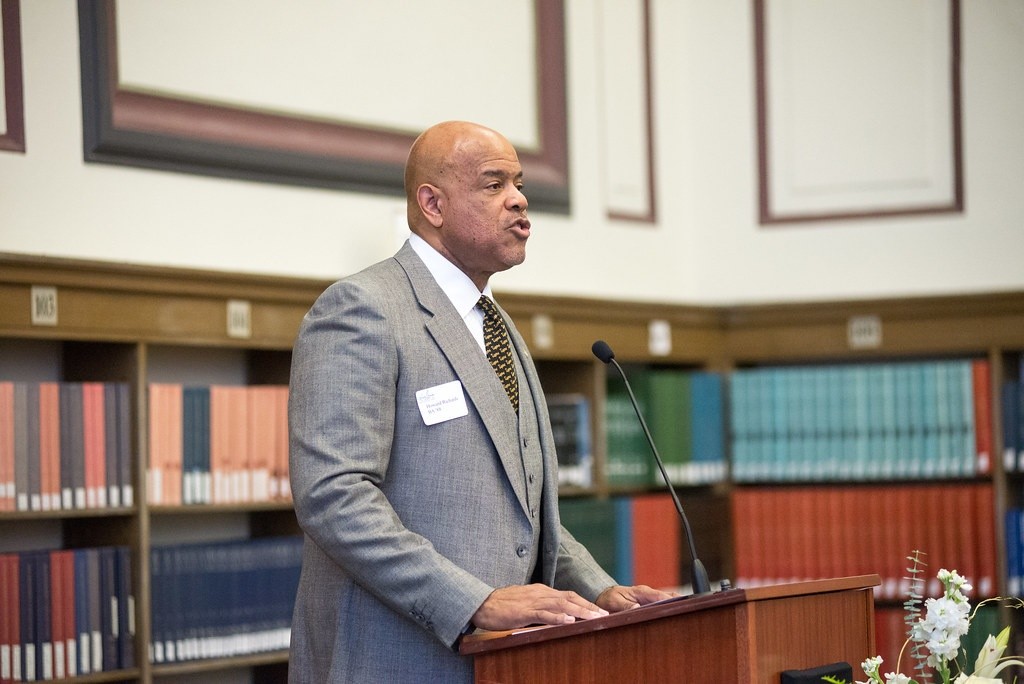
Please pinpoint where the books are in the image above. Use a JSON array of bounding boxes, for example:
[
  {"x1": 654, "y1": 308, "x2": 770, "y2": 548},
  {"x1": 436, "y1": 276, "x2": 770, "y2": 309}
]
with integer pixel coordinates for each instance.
[{"x1": 0, "y1": 358, "x2": 1023, "y2": 683}]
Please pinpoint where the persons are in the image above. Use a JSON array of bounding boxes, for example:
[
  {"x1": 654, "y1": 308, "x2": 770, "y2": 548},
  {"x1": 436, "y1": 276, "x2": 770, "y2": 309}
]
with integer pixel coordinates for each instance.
[{"x1": 288, "y1": 122, "x2": 679, "y2": 684}]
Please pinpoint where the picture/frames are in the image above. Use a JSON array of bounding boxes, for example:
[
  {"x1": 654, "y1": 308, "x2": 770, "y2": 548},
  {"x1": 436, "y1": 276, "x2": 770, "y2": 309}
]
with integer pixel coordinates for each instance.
[
  {"x1": 76, "y1": 1, "x2": 574, "y2": 213},
  {"x1": 751, "y1": 1, "x2": 963, "y2": 224},
  {"x1": 603, "y1": 1, "x2": 656, "y2": 222}
]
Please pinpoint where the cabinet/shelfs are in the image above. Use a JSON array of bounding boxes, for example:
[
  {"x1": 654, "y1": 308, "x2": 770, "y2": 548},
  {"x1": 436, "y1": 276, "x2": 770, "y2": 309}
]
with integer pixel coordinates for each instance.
[{"x1": 1, "y1": 250, "x2": 1024, "y2": 684}]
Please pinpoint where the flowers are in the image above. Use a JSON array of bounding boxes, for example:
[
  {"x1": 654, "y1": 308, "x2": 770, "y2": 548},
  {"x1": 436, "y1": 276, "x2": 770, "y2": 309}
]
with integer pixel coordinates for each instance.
[{"x1": 821, "y1": 548, "x2": 1023, "y2": 684}]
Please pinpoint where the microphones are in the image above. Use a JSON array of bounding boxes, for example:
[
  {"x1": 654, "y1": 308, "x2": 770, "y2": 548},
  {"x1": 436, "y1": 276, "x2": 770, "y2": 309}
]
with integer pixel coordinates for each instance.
[{"x1": 592, "y1": 340, "x2": 713, "y2": 598}]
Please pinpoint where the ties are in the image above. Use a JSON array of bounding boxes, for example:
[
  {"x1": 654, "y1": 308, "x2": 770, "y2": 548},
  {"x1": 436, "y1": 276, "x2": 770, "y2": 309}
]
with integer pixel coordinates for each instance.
[{"x1": 476, "y1": 295, "x2": 519, "y2": 420}]
